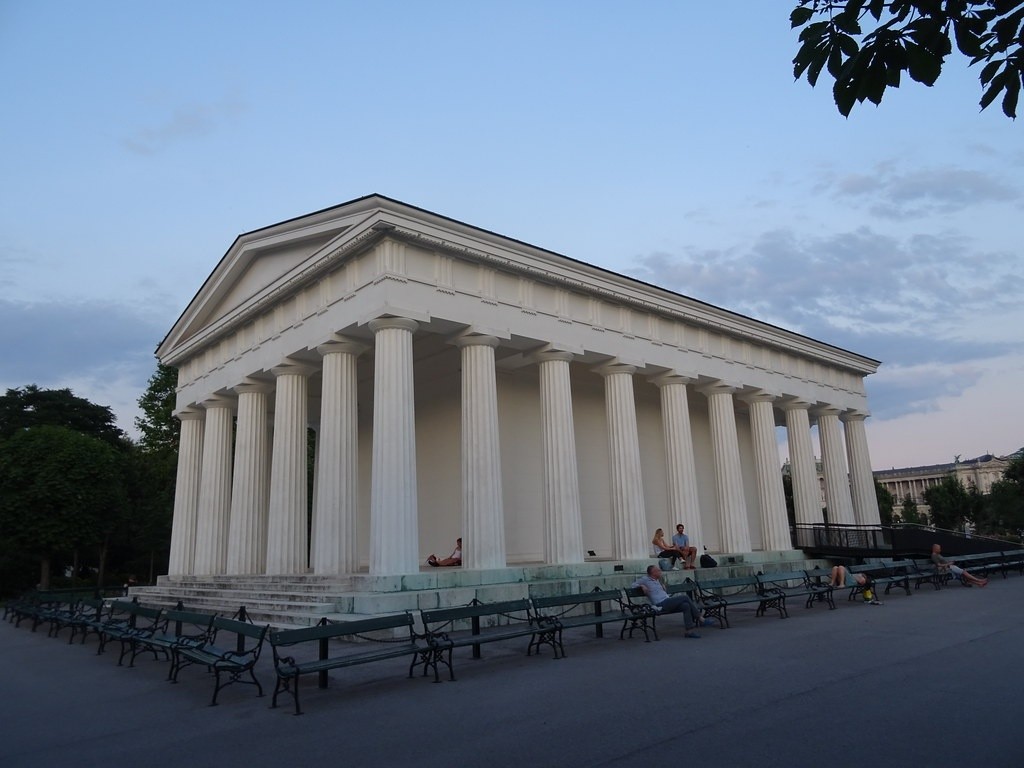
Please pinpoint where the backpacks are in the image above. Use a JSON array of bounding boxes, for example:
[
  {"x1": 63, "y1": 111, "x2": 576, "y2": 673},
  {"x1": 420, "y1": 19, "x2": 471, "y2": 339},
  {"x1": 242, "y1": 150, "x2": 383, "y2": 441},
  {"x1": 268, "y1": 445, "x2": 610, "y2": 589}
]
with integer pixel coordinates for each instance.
[{"x1": 700, "y1": 554, "x2": 717, "y2": 568}]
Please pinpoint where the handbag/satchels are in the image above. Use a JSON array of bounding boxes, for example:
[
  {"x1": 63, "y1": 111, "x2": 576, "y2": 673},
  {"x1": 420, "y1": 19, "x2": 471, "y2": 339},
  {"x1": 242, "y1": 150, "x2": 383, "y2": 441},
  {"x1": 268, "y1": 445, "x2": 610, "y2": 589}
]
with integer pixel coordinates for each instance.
[{"x1": 659, "y1": 559, "x2": 672, "y2": 571}]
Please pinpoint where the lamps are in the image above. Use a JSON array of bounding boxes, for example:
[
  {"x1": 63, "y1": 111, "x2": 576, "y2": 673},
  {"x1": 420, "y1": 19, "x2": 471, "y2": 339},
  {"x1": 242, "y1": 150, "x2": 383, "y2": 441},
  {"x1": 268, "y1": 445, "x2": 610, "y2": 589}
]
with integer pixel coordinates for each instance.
[{"x1": 588, "y1": 551, "x2": 596, "y2": 556}]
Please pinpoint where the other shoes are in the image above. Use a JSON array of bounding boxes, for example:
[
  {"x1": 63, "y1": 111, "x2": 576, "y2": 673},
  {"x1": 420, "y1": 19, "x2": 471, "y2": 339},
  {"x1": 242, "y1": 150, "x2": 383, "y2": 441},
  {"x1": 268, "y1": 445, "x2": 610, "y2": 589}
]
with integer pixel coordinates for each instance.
[
  {"x1": 684, "y1": 632, "x2": 701, "y2": 638},
  {"x1": 671, "y1": 566, "x2": 680, "y2": 571},
  {"x1": 680, "y1": 559, "x2": 686, "y2": 564},
  {"x1": 684, "y1": 565, "x2": 695, "y2": 569},
  {"x1": 700, "y1": 618, "x2": 715, "y2": 625}
]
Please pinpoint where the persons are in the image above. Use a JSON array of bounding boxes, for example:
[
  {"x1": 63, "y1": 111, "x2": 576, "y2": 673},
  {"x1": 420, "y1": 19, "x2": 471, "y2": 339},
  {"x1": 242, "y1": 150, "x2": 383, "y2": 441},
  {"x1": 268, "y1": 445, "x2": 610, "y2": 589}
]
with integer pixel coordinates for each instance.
[
  {"x1": 931, "y1": 544, "x2": 990, "y2": 586},
  {"x1": 826, "y1": 566, "x2": 870, "y2": 589},
  {"x1": 123, "y1": 575, "x2": 136, "y2": 596},
  {"x1": 428, "y1": 538, "x2": 462, "y2": 567},
  {"x1": 672, "y1": 524, "x2": 697, "y2": 569},
  {"x1": 652, "y1": 528, "x2": 686, "y2": 570},
  {"x1": 631, "y1": 565, "x2": 715, "y2": 638}
]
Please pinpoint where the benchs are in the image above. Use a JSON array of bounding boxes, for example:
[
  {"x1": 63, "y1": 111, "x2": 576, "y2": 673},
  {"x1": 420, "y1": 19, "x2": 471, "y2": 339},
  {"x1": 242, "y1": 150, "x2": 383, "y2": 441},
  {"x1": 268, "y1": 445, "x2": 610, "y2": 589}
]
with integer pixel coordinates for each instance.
[
  {"x1": 804, "y1": 565, "x2": 874, "y2": 609},
  {"x1": 528, "y1": 586, "x2": 651, "y2": 659},
  {"x1": 419, "y1": 596, "x2": 559, "y2": 682},
  {"x1": 622, "y1": 577, "x2": 725, "y2": 641},
  {"x1": 974, "y1": 552, "x2": 1009, "y2": 580},
  {"x1": 914, "y1": 559, "x2": 967, "y2": 591},
  {"x1": 175, "y1": 614, "x2": 268, "y2": 707},
  {"x1": 755, "y1": 570, "x2": 834, "y2": 619},
  {"x1": 267, "y1": 611, "x2": 443, "y2": 718},
  {"x1": 847, "y1": 562, "x2": 909, "y2": 601},
  {"x1": 1002, "y1": 550, "x2": 1024, "y2": 576},
  {"x1": 2, "y1": 589, "x2": 138, "y2": 666},
  {"x1": 123, "y1": 608, "x2": 218, "y2": 681},
  {"x1": 691, "y1": 576, "x2": 785, "y2": 628},
  {"x1": 945, "y1": 555, "x2": 988, "y2": 588},
  {"x1": 883, "y1": 559, "x2": 938, "y2": 595}
]
